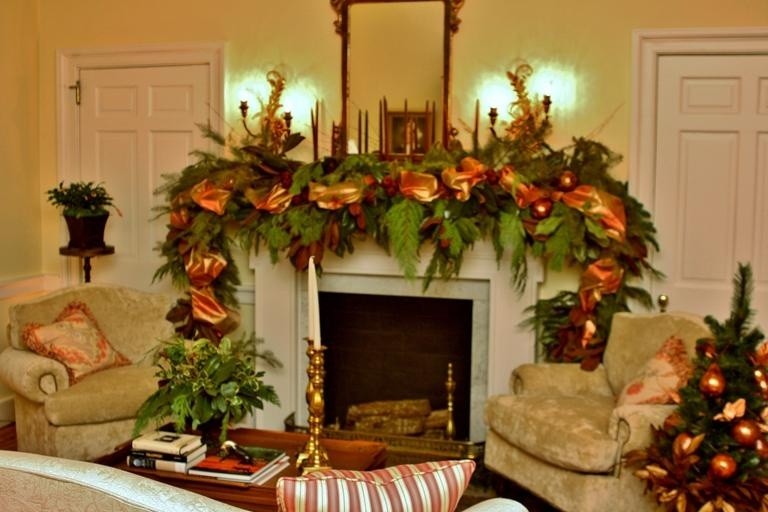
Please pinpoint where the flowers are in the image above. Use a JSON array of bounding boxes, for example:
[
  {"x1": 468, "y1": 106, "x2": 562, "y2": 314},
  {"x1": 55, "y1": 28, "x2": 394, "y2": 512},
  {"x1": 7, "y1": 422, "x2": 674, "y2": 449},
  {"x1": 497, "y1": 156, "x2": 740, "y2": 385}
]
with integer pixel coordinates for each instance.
[
  {"x1": 611, "y1": 262, "x2": 768, "y2": 512},
  {"x1": 151, "y1": 61, "x2": 667, "y2": 370}
]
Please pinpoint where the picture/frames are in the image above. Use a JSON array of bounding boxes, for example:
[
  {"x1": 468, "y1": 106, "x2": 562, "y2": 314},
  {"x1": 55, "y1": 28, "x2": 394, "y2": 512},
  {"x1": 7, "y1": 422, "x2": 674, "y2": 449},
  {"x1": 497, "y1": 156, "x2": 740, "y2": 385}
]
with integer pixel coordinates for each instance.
[{"x1": 383, "y1": 112, "x2": 435, "y2": 163}]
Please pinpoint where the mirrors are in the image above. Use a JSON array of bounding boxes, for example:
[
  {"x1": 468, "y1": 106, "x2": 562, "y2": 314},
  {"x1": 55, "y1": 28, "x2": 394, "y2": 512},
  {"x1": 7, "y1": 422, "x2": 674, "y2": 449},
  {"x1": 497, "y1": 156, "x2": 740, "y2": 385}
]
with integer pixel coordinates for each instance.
[{"x1": 329, "y1": 0, "x2": 467, "y2": 164}]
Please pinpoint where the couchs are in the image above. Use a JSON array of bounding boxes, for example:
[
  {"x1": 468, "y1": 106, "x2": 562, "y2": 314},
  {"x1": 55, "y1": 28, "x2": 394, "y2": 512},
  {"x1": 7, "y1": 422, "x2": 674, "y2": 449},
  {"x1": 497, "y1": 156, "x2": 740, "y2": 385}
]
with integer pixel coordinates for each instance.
[{"x1": 0, "y1": 450, "x2": 531, "y2": 512}]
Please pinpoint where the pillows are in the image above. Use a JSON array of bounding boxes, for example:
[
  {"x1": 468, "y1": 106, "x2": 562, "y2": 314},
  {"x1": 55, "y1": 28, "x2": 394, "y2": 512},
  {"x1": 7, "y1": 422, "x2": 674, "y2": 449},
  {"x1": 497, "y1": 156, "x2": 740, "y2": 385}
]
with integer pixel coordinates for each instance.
[
  {"x1": 21, "y1": 298, "x2": 131, "y2": 385},
  {"x1": 616, "y1": 331, "x2": 692, "y2": 407},
  {"x1": 276, "y1": 459, "x2": 477, "y2": 512}
]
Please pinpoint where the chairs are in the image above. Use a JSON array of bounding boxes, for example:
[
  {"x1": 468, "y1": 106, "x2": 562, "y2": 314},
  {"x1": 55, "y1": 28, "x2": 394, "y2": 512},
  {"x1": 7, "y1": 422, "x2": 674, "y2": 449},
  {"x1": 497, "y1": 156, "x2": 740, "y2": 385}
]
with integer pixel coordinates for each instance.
[
  {"x1": 484, "y1": 312, "x2": 714, "y2": 512},
  {"x1": 0, "y1": 283, "x2": 189, "y2": 462}
]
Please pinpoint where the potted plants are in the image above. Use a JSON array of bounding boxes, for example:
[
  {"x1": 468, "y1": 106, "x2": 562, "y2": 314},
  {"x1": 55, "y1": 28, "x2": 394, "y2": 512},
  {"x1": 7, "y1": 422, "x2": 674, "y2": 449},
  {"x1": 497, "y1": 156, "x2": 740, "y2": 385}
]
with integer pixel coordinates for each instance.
[
  {"x1": 131, "y1": 331, "x2": 284, "y2": 450},
  {"x1": 45, "y1": 180, "x2": 122, "y2": 249}
]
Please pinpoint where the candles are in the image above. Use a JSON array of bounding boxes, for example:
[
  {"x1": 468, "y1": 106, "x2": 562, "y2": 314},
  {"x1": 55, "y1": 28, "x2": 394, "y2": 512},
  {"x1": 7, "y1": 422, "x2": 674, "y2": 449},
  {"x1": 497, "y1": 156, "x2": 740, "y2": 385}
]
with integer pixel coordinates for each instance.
[{"x1": 306, "y1": 255, "x2": 321, "y2": 349}]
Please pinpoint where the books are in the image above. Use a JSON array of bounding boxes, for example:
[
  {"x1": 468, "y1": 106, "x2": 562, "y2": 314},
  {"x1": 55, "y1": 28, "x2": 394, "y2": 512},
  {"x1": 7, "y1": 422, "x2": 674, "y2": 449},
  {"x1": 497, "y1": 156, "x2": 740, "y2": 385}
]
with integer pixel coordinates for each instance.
[{"x1": 126, "y1": 429, "x2": 291, "y2": 488}]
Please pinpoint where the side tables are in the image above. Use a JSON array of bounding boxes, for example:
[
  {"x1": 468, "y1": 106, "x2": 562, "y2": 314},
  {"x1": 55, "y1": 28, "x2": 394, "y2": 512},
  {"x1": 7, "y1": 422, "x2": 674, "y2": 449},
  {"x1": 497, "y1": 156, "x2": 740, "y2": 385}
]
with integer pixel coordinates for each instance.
[{"x1": 57, "y1": 245, "x2": 116, "y2": 282}]
